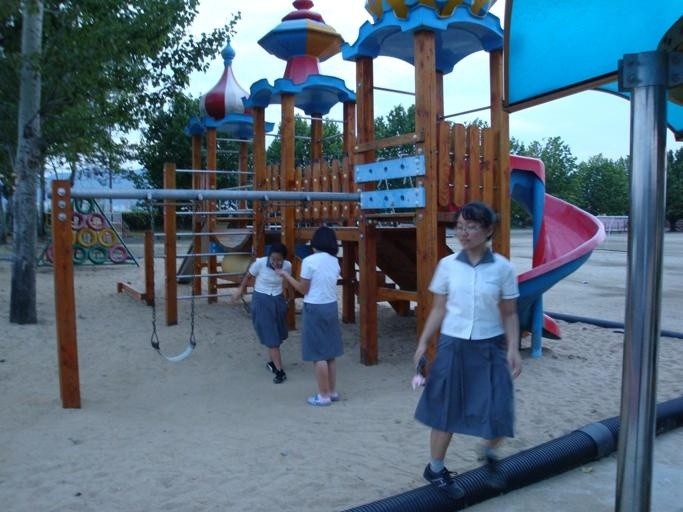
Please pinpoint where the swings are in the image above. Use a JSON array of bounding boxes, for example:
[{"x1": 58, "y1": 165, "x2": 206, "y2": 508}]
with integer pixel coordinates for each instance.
[
  {"x1": 146, "y1": 199, "x2": 200, "y2": 360},
  {"x1": 241, "y1": 201, "x2": 308, "y2": 317}
]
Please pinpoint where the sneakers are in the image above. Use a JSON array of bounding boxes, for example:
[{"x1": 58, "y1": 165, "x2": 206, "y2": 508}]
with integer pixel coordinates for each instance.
[
  {"x1": 307, "y1": 392, "x2": 338, "y2": 407},
  {"x1": 265, "y1": 361, "x2": 286, "y2": 383},
  {"x1": 423, "y1": 461, "x2": 465, "y2": 497}
]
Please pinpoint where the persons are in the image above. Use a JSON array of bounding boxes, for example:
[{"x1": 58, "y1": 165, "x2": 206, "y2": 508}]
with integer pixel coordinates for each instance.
[
  {"x1": 230, "y1": 244, "x2": 293, "y2": 387},
  {"x1": 275, "y1": 224, "x2": 342, "y2": 406},
  {"x1": 408, "y1": 200, "x2": 525, "y2": 500}
]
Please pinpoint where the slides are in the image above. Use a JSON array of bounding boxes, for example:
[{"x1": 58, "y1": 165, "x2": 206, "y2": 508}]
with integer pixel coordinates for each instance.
[{"x1": 509, "y1": 153, "x2": 605, "y2": 338}]
